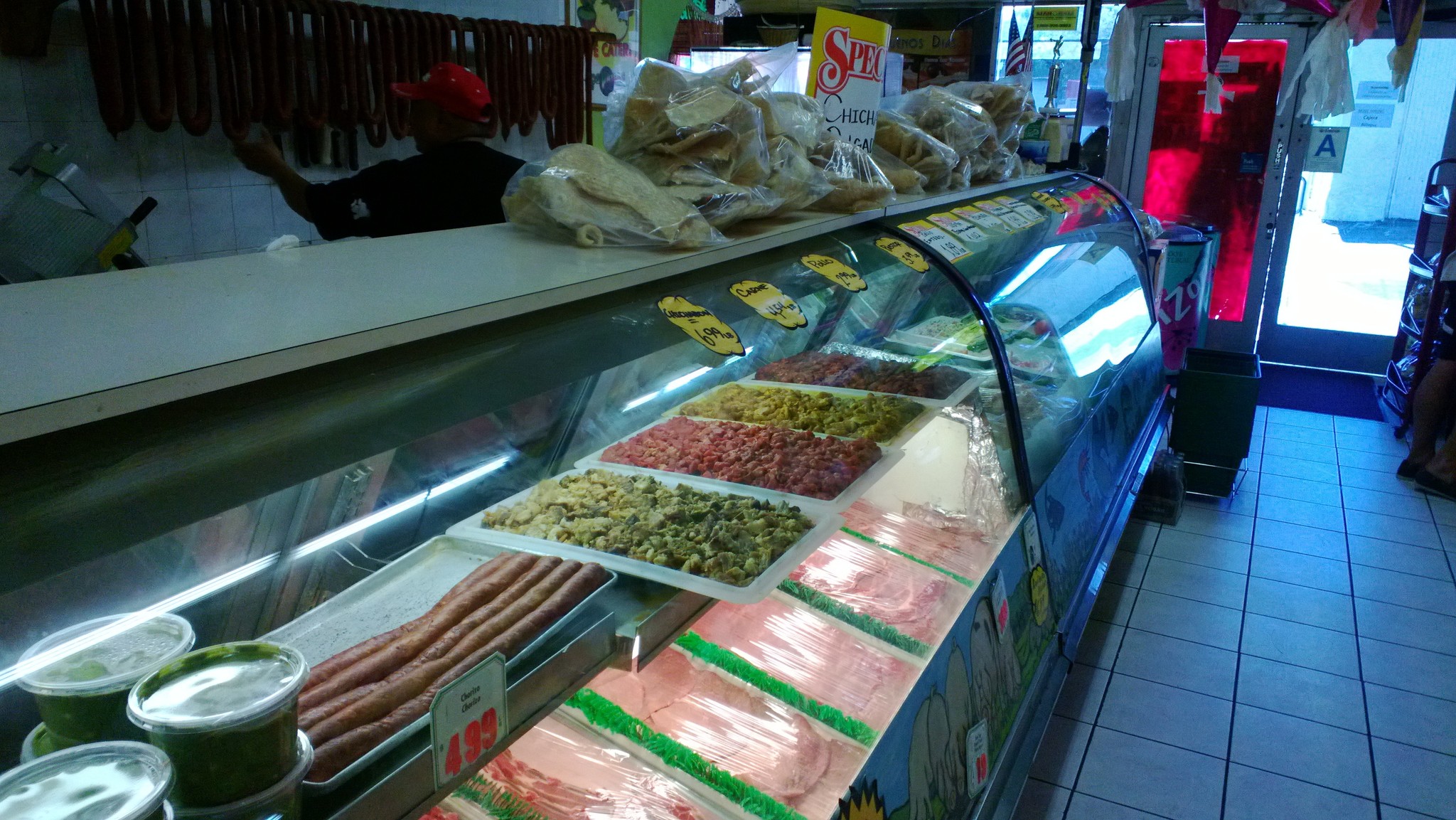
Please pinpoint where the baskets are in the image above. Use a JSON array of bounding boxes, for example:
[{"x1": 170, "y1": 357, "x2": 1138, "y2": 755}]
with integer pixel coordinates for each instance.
[{"x1": 757, "y1": 26, "x2": 800, "y2": 47}]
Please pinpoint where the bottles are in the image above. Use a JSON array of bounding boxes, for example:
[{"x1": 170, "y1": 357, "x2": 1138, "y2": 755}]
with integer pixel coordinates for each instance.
[{"x1": 1039, "y1": 112, "x2": 1067, "y2": 162}]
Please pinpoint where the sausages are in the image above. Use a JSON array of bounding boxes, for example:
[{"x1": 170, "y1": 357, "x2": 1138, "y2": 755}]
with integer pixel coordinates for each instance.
[
  {"x1": 0, "y1": 0, "x2": 594, "y2": 170},
  {"x1": 295, "y1": 550, "x2": 609, "y2": 787}
]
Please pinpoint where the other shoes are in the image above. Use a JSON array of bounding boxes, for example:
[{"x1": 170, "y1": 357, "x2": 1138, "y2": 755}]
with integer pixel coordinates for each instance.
[
  {"x1": 1396, "y1": 460, "x2": 1421, "y2": 481},
  {"x1": 1414, "y1": 468, "x2": 1455, "y2": 501}
]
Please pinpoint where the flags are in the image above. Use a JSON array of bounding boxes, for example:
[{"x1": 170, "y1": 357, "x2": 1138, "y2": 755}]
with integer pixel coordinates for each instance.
[{"x1": 1006, "y1": 7, "x2": 1034, "y2": 92}]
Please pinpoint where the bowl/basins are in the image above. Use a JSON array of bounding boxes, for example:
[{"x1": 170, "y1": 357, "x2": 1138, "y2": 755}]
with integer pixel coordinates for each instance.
[{"x1": 0, "y1": 611, "x2": 316, "y2": 820}]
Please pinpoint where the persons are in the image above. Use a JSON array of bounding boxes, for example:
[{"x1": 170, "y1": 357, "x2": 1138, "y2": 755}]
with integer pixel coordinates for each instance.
[
  {"x1": 1081, "y1": 123, "x2": 1110, "y2": 179},
  {"x1": 1395, "y1": 247, "x2": 1456, "y2": 501},
  {"x1": 240, "y1": 62, "x2": 530, "y2": 242}
]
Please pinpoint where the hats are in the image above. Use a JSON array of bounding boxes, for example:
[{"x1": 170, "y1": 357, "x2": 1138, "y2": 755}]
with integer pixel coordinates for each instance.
[{"x1": 391, "y1": 62, "x2": 492, "y2": 124}]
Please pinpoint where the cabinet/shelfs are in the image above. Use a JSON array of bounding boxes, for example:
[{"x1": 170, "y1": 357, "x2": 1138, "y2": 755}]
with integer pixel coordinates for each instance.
[{"x1": 1382, "y1": 157, "x2": 1456, "y2": 438}]
